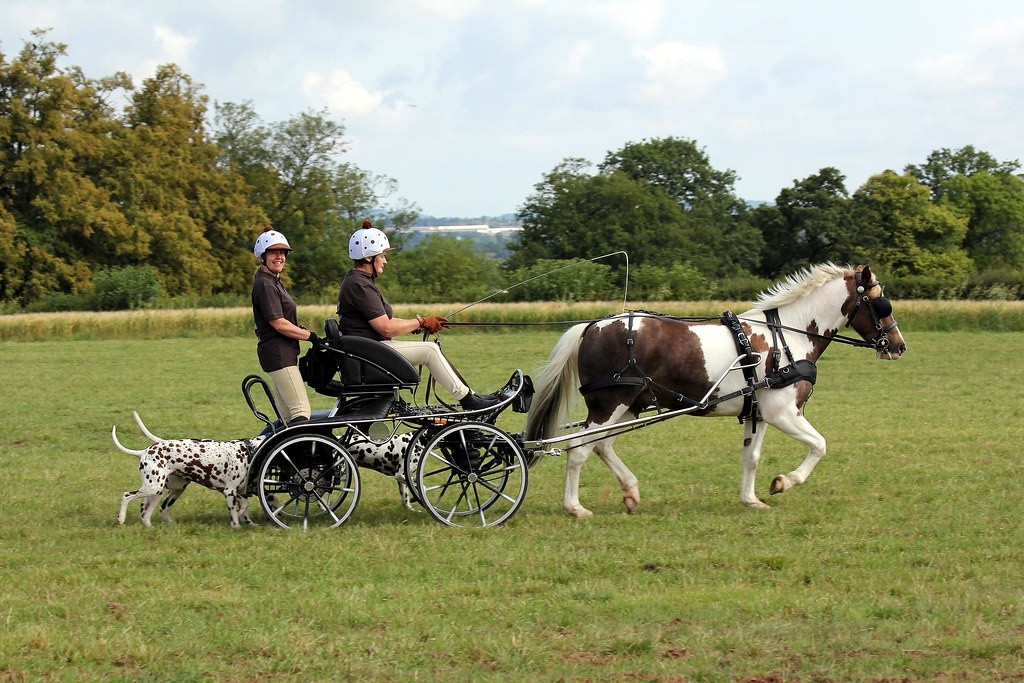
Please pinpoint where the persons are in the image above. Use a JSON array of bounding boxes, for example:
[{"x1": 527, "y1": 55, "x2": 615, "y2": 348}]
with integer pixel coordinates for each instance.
[
  {"x1": 250, "y1": 226, "x2": 326, "y2": 490},
  {"x1": 335, "y1": 220, "x2": 502, "y2": 421}
]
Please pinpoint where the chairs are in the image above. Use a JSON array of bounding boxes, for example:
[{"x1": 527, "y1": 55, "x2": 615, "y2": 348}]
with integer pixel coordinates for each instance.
[{"x1": 323, "y1": 317, "x2": 421, "y2": 397}]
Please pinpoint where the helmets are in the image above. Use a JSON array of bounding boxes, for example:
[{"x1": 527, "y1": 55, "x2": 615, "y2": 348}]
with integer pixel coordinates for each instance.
[
  {"x1": 348, "y1": 220, "x2": 397, "y2": 261},
  {"x1": 254, "y1": 225, "x2": 294, "y2": 260}
]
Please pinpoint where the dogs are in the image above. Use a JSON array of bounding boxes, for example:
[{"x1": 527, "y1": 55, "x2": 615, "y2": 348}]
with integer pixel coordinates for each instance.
[
  {"x1": 304, "y1": 428, "x2": 448, "y2": 513},
  {"x1": 133, "y1": 410, "x2": 281, "y2": 523},
  {"x1": 112, "y1": 425, "x2": 273, "y2": 528}
]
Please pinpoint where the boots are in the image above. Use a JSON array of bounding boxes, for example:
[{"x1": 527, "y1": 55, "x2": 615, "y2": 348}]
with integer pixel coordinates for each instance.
[{"x1": 461, "y1": 390, "x2": 500, "y2": 420}]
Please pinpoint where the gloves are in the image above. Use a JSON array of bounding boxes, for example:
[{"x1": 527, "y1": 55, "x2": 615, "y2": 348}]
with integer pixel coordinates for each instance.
[
  {"x1": 307, "y1": 331, "x2": 323, "y2": 348},
  {"x1": 419, "y1": 313, "x2": 449, "y2": 332}
]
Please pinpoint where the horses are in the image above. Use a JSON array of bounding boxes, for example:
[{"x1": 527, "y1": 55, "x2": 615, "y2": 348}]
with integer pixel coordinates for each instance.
[{"x1": 519, "y1": 259, "x2": 907, "y2": 518}]
